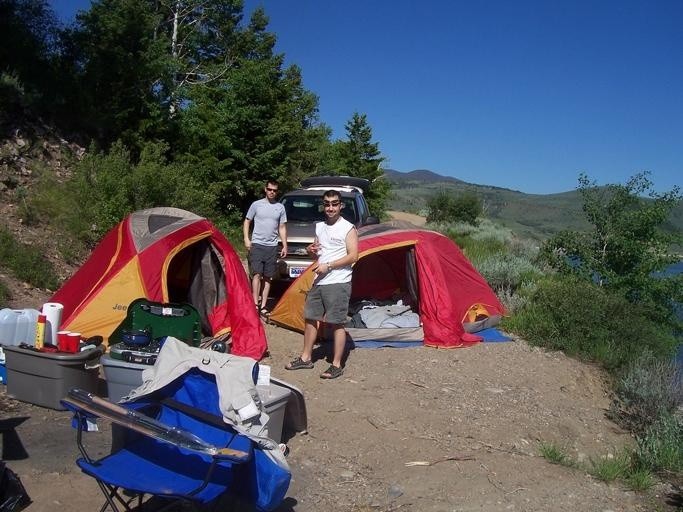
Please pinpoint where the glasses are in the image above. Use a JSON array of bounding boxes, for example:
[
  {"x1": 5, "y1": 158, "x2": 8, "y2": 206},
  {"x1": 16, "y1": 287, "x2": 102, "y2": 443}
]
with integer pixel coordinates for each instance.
[
  {"x1": 322, "y1": 200, "x2": 340, "y2": 207},
  {"x1": 267, "y1": 188, "x2": 278, "y2": 192}
]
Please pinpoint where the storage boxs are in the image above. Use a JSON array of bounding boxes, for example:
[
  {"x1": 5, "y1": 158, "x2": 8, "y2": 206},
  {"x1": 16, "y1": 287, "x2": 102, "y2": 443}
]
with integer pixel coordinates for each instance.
[
  {"x1": 5, "y1": 342, "x2": 106, "y2": 408},
  {"x1": 101, "y1": 349, "x2": 294, "y2": 445}
]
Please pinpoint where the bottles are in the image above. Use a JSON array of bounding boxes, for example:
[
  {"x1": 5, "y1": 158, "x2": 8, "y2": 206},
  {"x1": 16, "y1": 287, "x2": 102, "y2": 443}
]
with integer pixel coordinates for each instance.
[{"x1": 35, "y1": 314, "x2": 46, "y2": 349}]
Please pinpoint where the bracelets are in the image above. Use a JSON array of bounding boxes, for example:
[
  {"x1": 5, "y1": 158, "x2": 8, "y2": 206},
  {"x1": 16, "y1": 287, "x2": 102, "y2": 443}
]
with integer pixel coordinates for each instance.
[{"x1": 327, "y1": 262, "x2": 331, "y2": 272}]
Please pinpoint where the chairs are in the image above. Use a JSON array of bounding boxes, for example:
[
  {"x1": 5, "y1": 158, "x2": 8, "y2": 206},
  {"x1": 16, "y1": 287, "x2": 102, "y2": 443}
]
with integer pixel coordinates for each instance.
[{"x1": 60, "y1": 338, "x2": 256, "y2": 512}]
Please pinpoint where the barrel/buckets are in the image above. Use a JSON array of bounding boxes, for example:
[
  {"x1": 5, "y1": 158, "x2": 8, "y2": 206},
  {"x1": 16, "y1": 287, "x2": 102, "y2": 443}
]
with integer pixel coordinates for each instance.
[{"x1": 1, "y1": 308, "x2": 43, "y2": 347}]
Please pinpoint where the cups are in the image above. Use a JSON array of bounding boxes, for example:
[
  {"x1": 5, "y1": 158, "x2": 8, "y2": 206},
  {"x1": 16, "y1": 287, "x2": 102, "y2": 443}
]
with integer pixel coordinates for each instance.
[
  {"x1": 67, "y1": 333, "x2": 81, "y2": 353},
  {"x1": 56, "y1": 331, "x2": 71, "y2": 352}
]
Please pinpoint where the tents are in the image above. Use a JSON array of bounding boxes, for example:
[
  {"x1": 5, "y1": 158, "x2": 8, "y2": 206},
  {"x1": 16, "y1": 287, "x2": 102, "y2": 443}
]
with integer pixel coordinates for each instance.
[
  {"x1": 266, "y1": 219, "x2": 515, "y2": 349},
  {"x1": 39, "y1": 206, "x2": 268, "y2": 363}
]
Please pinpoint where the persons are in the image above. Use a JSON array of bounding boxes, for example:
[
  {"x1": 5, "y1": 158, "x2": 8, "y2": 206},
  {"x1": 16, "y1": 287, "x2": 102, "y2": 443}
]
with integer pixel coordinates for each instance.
[
  {"x1": 284, "y1": 189, "x2": 359, "y2": 380},
  {"x1": 242, "y1": 179, "x2": 289, "y2": 318}
]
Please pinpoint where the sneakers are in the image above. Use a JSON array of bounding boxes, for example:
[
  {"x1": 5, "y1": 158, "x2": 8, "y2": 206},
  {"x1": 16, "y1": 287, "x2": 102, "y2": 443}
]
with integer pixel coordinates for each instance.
[
  {"x1": 321, "y1": 365, "x2": 344, "y2": 379},
  {"x1": 285, "y1": 358, "x2": 314, "y2": 370}
]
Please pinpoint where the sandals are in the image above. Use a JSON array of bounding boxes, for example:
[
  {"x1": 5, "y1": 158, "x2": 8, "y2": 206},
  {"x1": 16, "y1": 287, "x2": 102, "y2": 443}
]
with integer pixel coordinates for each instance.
[{"x1": 256, "y1": 305, "x2": 270, "y2": 318}]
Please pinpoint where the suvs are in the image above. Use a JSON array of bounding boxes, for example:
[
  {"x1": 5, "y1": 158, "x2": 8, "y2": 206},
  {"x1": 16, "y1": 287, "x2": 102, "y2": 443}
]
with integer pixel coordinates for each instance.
[{"x1": 249, "y1": 172, "x2": 377, "y2": 291}]
